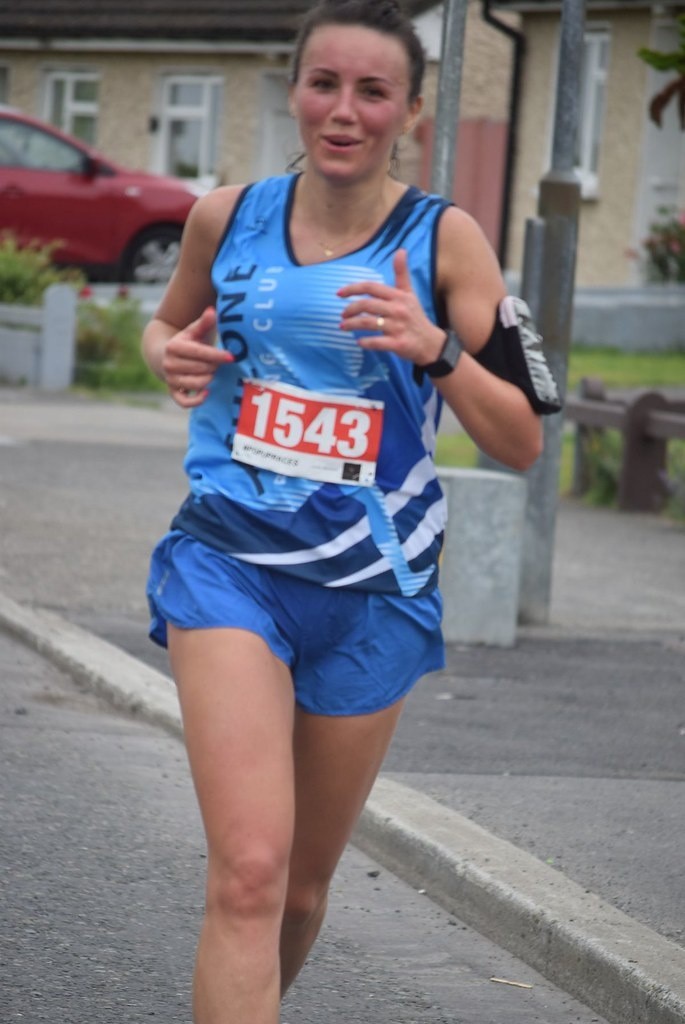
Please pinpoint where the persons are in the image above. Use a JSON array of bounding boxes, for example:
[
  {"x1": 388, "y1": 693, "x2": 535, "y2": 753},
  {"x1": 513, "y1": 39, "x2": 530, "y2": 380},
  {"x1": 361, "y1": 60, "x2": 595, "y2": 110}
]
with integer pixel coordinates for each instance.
[{"x1": 144, "y1": 0, "x2": 547, "y2": 1024}]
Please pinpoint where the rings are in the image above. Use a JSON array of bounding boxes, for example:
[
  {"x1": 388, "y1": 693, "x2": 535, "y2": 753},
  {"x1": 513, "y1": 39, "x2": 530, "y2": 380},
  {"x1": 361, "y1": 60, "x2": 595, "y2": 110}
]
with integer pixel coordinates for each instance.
[{"x1": 377, "y1": 317, "x2": 384, "y2": 328}]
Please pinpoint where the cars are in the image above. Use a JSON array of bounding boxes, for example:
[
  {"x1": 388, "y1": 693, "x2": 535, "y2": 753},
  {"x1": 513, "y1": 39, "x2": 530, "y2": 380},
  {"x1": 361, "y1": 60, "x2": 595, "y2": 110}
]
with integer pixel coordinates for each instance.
[{"x1": 0, "y1": 107, "x2": 223, "y2": 294}]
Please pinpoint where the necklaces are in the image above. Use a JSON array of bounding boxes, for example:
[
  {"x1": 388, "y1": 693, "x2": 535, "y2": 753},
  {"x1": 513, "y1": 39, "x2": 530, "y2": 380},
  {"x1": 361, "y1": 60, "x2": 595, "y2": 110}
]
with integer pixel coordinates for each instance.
[{"x1": 309, "y1": 224, "x2": 358, "y2": 258}]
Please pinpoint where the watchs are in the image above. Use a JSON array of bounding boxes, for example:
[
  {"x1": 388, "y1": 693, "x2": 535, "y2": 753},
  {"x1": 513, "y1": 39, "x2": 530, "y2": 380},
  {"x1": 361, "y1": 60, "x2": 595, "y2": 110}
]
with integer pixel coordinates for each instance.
[{"x1": 412, "y1": 329, "x2": 465, "y2": 388}]
